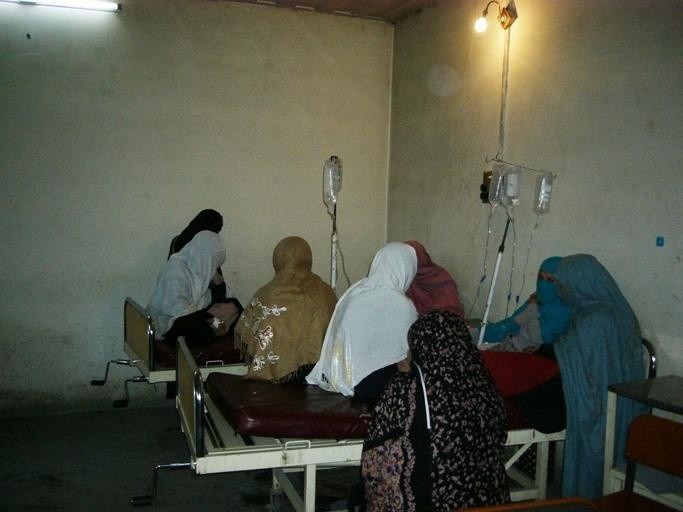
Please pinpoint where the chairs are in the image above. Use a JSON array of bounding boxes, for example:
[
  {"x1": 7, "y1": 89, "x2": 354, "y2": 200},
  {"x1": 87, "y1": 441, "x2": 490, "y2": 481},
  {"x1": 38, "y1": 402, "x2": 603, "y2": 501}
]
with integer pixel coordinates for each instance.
[{"x1": 591, "y1": 414, "x2": 683, "y2": 512}]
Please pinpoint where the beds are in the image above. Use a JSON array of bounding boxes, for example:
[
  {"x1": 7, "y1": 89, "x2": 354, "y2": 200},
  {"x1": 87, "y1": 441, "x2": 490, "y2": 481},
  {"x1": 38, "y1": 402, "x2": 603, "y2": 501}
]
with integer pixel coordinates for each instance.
[
  {"x1": 90, "y1": 296, "x2": 492, "y2": 409},
  {"x1": 128, "y1": 335, "x2": 657, "y2": 511}
]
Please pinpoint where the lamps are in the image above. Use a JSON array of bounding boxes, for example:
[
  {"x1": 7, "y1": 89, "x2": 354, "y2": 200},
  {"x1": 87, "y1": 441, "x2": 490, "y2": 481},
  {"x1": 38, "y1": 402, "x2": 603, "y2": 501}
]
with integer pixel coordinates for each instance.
[
  {"x1": 1, "y1": 0, "x2": 122, "y2": 13},
  {"x1": 475, "y1": 2, "x2": 516, "y2": 32}
]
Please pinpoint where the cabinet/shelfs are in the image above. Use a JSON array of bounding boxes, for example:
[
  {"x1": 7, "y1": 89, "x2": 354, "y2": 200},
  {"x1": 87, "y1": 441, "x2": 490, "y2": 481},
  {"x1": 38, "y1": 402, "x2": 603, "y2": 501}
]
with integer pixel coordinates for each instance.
[{"x1": 603, "y1": 375, "x2": 683, "y2": 512}]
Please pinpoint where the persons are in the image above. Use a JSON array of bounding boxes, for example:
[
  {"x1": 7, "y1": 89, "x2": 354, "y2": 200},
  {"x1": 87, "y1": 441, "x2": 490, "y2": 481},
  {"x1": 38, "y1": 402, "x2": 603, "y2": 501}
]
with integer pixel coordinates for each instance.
[
  {"x1": 360, "y1": 310, "x2": 512, "y2": 511},
  {"x1": 234, "y1": 236, "x2": 338, "y2": 386},
  {"x1": 404, "y1": 240, "x2": 464, "y2": 318},
  {"x1": 149, "y1": 209, "x2": 243, "y2": 351},
  {"x1": 302, "y1": 241, "x2": 421, "y2": 411},
  {"x1": 465, "y1": 253, "x2": 646, "y2": 499}
]
